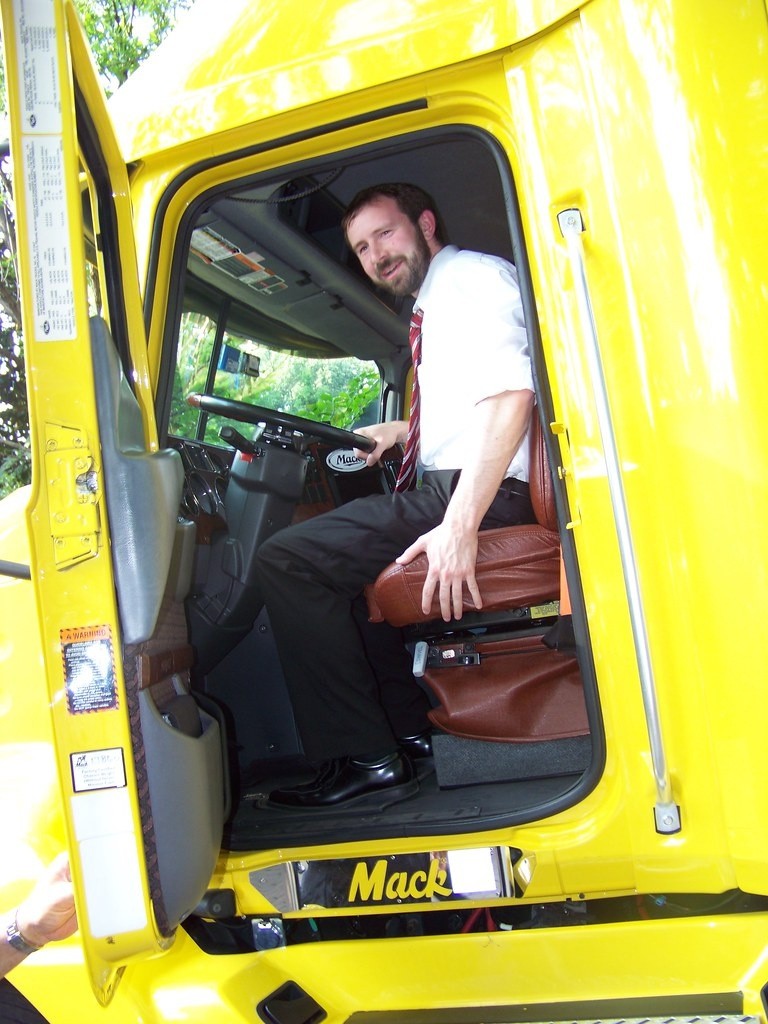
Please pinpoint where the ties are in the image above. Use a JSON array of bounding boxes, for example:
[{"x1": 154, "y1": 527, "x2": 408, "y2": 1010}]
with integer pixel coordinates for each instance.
[{"x1": 395, "y1": 308, "x2": 424, "y2": 493}]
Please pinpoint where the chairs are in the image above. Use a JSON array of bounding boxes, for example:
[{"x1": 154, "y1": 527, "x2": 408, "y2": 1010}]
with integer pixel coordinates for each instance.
[{"x1": 365, "y1": 402, "x2": 591, "y2": 741}]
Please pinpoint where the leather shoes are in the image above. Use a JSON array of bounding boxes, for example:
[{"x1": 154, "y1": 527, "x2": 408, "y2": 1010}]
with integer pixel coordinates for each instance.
[{"x1": 264, "y1": 728, "x2": 436, "y2": 814}]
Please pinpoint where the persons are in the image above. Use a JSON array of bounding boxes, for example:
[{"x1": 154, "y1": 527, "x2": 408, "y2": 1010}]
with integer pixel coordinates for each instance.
[
  {"x1": 259, "y1": 183, "x2": 536, "y2": 810},
  {"x1": 0, "y1": 850, "x2": 79, "y2": 977}
]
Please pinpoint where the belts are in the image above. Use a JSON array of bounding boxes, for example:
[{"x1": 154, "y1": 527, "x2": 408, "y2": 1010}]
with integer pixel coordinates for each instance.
[{"x1": 500, "y1": 477, "x2": 531, "y2": 498}]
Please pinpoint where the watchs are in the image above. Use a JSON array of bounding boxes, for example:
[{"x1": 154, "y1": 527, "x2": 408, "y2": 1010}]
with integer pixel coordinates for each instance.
[{"x1": 6, "y1": 908, "x2": 42, "y2": 955}]
